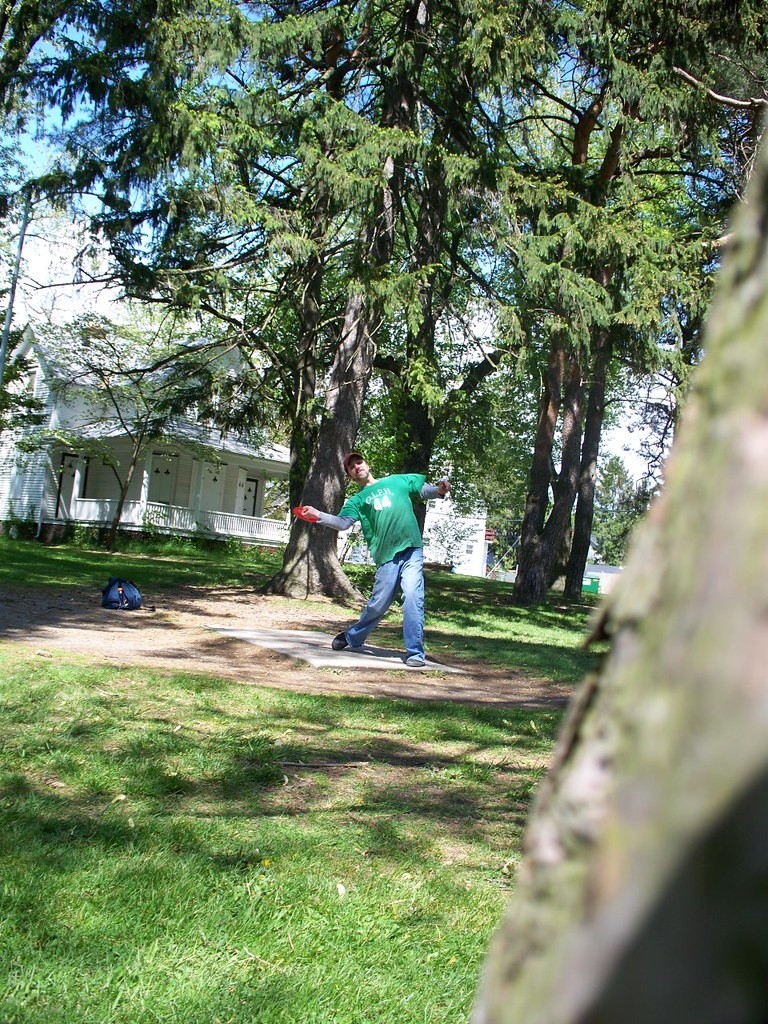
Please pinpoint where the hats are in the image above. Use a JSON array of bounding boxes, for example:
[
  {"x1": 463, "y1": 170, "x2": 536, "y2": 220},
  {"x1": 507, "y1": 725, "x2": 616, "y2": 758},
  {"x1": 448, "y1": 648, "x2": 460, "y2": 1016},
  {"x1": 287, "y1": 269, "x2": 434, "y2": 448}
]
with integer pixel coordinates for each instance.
[{"x1": 342, "y1": 451, "x2": 362, "y2": 465}]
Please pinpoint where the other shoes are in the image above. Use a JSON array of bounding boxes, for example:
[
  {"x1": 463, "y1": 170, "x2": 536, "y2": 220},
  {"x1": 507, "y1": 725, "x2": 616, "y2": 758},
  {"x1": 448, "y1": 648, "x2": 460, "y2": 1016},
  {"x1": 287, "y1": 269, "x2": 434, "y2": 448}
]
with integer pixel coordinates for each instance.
[
  {"x1": 332, "y1": 632, "x2": 348, "y2": 650},
  {"x1": 406, "y1": 657, "x2": 426, "y2": 667}
]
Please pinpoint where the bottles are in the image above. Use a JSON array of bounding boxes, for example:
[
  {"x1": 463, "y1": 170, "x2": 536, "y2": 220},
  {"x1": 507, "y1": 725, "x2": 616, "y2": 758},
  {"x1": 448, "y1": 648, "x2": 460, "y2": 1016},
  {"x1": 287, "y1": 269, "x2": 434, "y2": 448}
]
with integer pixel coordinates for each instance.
[{"x1": 118, "y1": 588, "x2": 123, "y2": 605}]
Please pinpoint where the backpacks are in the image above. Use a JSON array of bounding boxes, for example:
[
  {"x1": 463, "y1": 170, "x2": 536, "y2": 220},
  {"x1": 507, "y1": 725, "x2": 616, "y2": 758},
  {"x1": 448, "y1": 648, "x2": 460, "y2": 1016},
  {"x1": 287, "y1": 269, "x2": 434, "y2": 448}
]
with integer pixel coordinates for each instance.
[{"x1": 101, "y1": 577, "x2": 142, "y2": 609}]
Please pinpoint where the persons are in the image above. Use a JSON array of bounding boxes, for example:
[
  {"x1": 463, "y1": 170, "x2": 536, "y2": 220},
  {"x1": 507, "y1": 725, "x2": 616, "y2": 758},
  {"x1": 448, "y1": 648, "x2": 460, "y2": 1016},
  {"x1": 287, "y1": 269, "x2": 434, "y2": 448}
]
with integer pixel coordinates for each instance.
[{"x1": 301, "y1": 454, "x2": 452, "y2": 667}]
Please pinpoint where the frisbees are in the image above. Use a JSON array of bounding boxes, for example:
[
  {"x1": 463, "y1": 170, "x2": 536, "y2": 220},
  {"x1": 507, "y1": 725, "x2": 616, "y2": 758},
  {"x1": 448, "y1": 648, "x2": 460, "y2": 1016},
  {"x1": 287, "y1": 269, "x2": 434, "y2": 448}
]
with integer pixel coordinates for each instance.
[{"x1": 292, "y1": 507, "x2": 318, "y2": 522}]
